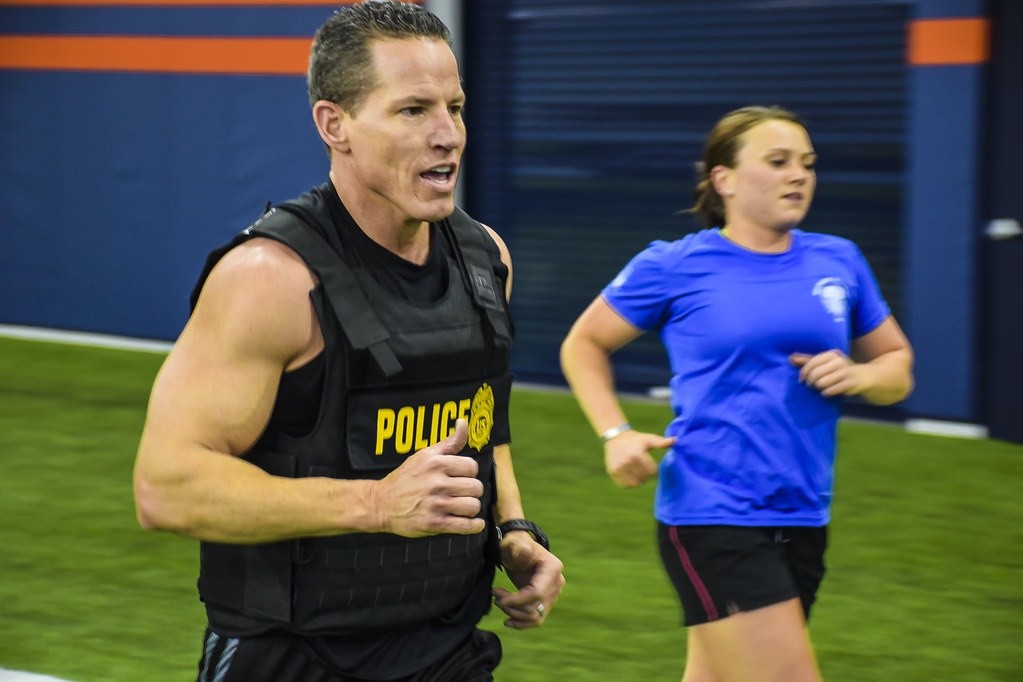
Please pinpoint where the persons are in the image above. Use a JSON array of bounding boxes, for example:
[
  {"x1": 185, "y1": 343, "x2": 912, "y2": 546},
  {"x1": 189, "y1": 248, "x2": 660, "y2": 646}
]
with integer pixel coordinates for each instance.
[
  {"x1": 558, "y1": 103, "x2": 914, "y2": 680},
  {"x1": 133, "y1": 1, "x2": 566, "y2": 682}
]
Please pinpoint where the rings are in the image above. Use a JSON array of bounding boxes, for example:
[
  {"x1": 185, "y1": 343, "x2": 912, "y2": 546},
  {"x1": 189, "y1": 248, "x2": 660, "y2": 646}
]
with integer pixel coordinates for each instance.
[{"x1": 536, "y1": 604, "x2": 545, "y2": 616}]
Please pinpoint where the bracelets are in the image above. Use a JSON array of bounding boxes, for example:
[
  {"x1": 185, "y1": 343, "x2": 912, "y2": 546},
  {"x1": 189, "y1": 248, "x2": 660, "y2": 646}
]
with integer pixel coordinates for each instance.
[
  {"x1": 496, "y1": 518, "x2": 550, "y2": 571},
  {"x1": 600, "y1": 423, "x2": 631, "y2": 445}
]
